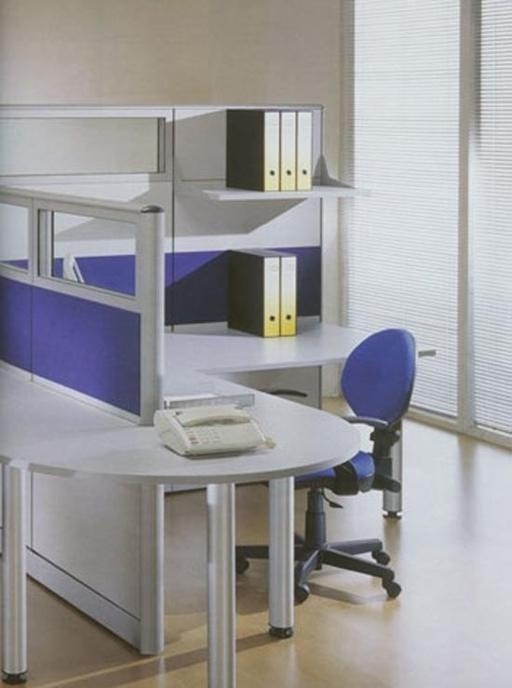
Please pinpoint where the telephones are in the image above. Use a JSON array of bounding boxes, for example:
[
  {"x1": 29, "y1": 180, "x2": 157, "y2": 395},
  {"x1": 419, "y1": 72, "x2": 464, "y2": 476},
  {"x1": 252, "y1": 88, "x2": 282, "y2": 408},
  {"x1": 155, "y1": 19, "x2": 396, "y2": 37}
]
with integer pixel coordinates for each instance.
[{"x1": 154, "y1": 404, "x2": 267, "y2": 456}]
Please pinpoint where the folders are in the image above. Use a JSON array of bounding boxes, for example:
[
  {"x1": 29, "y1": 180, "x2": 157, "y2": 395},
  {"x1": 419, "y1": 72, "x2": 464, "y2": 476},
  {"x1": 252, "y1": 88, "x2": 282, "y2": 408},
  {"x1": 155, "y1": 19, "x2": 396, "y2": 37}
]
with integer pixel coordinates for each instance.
[
  {"x1": 227, "y1": 248, "x2": 297, "y2": 338},
  {"x1": 226, "y1": 108, "x2": 311, "y2": 191}
]
